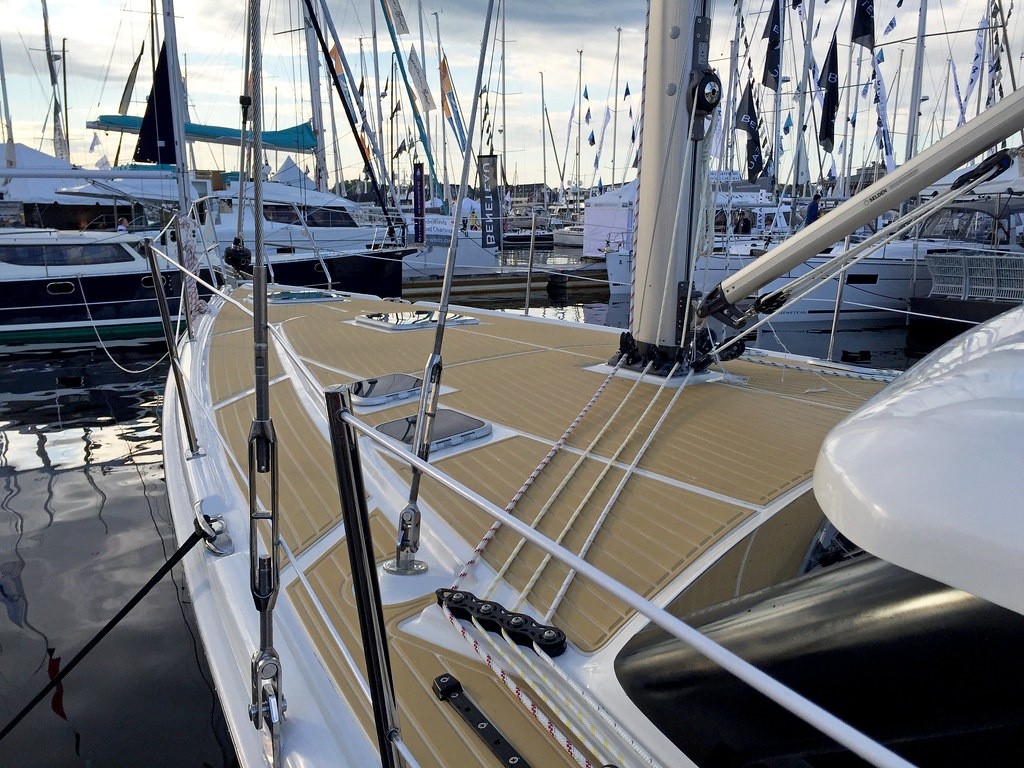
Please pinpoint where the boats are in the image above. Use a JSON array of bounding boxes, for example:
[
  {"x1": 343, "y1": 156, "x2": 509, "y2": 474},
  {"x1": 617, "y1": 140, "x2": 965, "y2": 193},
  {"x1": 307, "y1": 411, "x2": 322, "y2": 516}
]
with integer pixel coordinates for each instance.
[
  {"x1": 0, "y1": 0, "x2": 1024, "y2": 370},
  {"x1": 134, "y1": 0, "x2": 1024, "y2": 768}
]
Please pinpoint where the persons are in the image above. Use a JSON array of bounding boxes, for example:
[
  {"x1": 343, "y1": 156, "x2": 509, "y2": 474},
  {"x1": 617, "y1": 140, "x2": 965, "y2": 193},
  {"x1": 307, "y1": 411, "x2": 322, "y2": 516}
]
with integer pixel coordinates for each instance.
[
  {"x1": 805, "y1": 194, "x2": 823, "y2": 228},
  {"x1": 729, "y1": 211, "x2": 775, "y2": 235},
  {"x1": 116, "y1": 217, "x2": 130, "y2": 233},
  {"x1": 470, "y1": 210, "x2": 481, "y2": 231},
  {"x1": 72, "y1": 208, "x2": 96, "y2": 231}
]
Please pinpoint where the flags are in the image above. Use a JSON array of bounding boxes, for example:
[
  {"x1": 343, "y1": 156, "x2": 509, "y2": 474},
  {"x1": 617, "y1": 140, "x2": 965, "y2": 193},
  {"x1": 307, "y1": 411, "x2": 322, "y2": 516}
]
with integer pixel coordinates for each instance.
[
  {"x1": 714, "y1": 0, "x2": 1024, "y2": 185},
  {"x1": 178, "y1": 0, "x2": 643, "y2": 224},
  {"x1": 0, "y1": 0, "x2": 178, "y2": 184}
]
